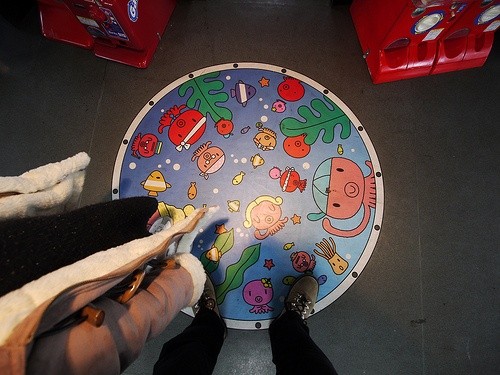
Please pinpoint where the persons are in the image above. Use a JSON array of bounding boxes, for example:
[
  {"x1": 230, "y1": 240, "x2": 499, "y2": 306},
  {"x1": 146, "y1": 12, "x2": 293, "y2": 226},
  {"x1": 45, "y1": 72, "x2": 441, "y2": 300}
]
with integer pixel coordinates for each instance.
[
  {"x1": 153, "y1": 271, "x2": 339, "y2": 374},
  {"x1": 0, "y1": 152, "x2": 219, "y2": 374}
]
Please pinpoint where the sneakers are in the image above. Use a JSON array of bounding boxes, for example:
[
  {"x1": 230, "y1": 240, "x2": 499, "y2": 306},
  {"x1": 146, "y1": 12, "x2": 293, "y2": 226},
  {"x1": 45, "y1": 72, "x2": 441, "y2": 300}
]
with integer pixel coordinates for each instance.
[
  {"x1": 148, "y1": 217, "x2": 173, "y2": 235},
  {"x1": 192, "y1": 272, "x2": 228, "y2": 339},
  {"x1": 145, "y1": 240, "x2": 178, "y2": 273},
  {"x1": 274, "y1": 275, "x2": 319, "y2": 325}
]
